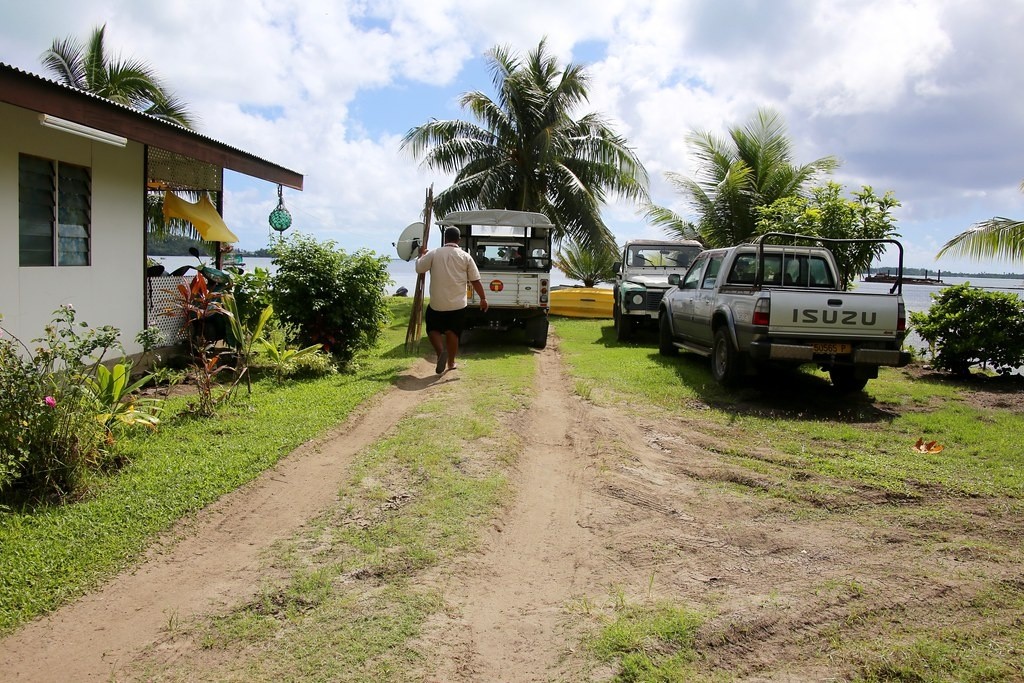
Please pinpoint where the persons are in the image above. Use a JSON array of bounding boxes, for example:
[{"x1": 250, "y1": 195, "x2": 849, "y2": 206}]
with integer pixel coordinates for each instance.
[
  {"x1": 513, "y1": 246, "x2": 526, "y2": 267},
  {"x1": 415, "y1": 226, "x2": 489, "y2": 374},
  {"x1": 477, "y1": 245, "x2": 490, "y2": 265}
]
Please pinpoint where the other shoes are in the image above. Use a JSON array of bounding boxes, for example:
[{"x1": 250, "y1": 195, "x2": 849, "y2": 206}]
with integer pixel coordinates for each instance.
[
  {"x1": 449, "y1": 362, "x2": 457, "y2": 369},
  {"x1": 436, "y1": 350, "x2": 448, "y2": 374}
]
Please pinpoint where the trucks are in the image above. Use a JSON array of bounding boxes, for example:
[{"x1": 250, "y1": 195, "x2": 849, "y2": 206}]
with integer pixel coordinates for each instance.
[{"x1": 433, "y1": 208, "x2": 555, "y2": 349}]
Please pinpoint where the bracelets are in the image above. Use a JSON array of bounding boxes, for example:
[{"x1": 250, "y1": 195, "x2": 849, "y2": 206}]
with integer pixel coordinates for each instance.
[{"x1": 481, "y1": 297, "x2": 487, "y2": 299}]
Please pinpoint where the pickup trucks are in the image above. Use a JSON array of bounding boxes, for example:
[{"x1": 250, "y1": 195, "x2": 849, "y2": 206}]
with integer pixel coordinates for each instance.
[
  {"x1": 658, "y1": 230, "x2": 914, "y2": 402},
  {"x1": 612, "y1": 239, "x2": 702, "y2": 342}
]
